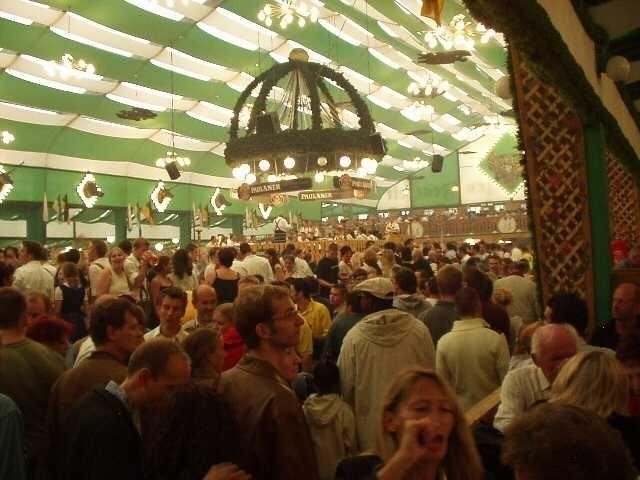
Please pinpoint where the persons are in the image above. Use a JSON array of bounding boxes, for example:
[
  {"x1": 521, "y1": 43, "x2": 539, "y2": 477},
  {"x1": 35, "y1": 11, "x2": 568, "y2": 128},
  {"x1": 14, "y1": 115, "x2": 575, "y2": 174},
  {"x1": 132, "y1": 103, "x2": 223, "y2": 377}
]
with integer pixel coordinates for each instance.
[{"x1": 1, "y1": 205, "x2": 640, "y2": 480}]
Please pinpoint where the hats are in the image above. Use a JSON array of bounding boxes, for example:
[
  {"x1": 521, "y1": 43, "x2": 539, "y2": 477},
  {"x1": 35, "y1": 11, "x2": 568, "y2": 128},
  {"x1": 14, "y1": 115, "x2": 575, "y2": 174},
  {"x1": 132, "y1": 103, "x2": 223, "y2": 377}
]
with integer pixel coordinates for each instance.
[{"x1": 352, "y1": 277, "x2": 395, "y2": 300}]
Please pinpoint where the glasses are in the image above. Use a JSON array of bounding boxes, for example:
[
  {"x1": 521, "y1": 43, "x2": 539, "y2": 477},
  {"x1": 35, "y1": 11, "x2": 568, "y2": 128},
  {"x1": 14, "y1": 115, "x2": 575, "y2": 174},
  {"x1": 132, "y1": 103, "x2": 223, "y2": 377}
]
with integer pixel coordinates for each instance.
[{"x1": 272, "y1": 311, "x2": 299, "y2": 321}]
